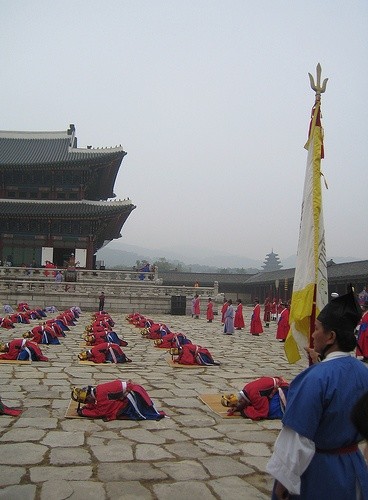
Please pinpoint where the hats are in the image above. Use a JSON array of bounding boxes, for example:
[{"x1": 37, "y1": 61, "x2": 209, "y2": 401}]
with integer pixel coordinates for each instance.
[
  {"x1": 1, "y1": 308, "x2": 67, "y2": 351},
  {"x1": 84, "y1": 335, "x2": 93, "y2": 342},
  {"x1": 126, "y1": 315, "x2": 162, "y2": 345},
  {"x1": 317, "y1": 292, "x2": 367, "y2": 362},
  {"x1": 78, "y1": 351, "x2": 87, "y2": 360},
  {"x1": 85, "y1": 312, "x2": 95, "y2": 332},
  {"x1": 168, "y1": 347, "x2": 178, "y2": 354},
  {"x1": 72, "y1": 386, "x2": 87, "y2": 403},
  {"x1": 221, "y1": 393, "x2": 238, "y2": 407},
  {"x1": 197, "y1": 292, "x2": 288, "y2": 307}
]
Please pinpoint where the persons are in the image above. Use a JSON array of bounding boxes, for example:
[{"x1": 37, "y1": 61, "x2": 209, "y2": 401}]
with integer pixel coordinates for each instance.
[
  {"x1": 265, "y1": 297, "x2": 288, "y2": 342},
  {"x1": 221, "y1": 376, "x2": 290, "y2": 420},
  {"x1": 221, "y1": 298, "x2": 235, "y2": 334},
  {"x1": 267, "y1": 284, "x2": 368, "y2": 500},
  {"x1": 84, "y1": 311, "x2": 128, "y2": 346},
  {"x1": 126, "y1": 312, "x2": 193, "y2": 347},
  {"x1": 70, "y1": 380, "x2": 165, "y2": 422},
  {"x1": 206, "y1": 297, "x2": 214, "y2": 323},
  {"x1": 0, "y1": 302, "x2": 59, "y2": 329},
  {"x1": 167, "y1": 344, "x2": 221, "y2": 366},
  {"x1": 99, "y1": 292, "x2": 105, "y2": 311},
  {"x1": 77, "y1": 342, "x2": 132, "y2": 364},
  {"x1": 192, "y1": 293, "x2": 201, "y2": 319},
  {"x1": 0, "y1": 339, "x2": 48, "y2": 362},
  {"x1": 23, "y1": 307, "x2": 82, "y2": 344},
  {"x1": 234, "y1": 299, "x2": 245, "y2": 330},
  {"x1": 250, "y1": 300, "x2": 264, "y2": 336}
]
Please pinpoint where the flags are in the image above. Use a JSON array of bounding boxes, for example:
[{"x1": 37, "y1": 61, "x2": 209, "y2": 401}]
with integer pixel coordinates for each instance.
[{"x1": 284, "y1": 106, "x2": 324, "y2": 368}]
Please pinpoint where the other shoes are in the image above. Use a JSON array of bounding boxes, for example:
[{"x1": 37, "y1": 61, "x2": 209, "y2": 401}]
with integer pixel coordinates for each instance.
[{"x1": 192, "y1": 316, "x2": 287, "y2": 342}]
